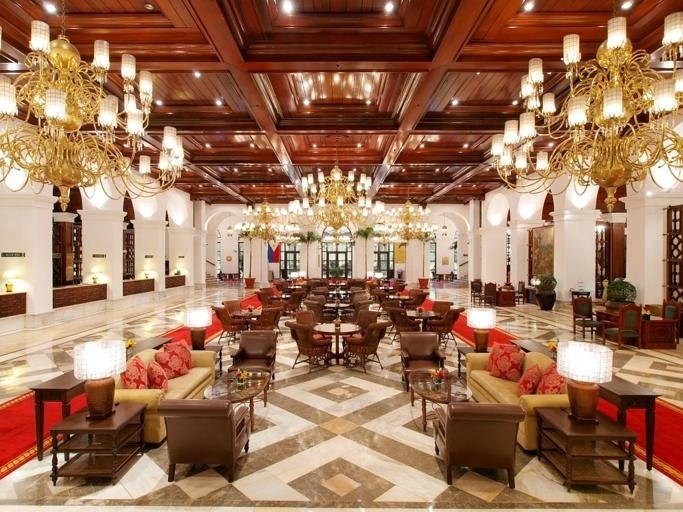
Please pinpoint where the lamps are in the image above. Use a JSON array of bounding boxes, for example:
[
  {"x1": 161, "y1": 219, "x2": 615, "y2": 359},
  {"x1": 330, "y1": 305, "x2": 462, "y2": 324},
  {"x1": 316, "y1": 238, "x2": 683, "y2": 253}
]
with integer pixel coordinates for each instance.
[
  {"x1": 0, "y1": 1, "x2": 186, "y2": 214},
  {"x1": 485, "y1": 1, "x2": 683, "y2": 216},
  {"x1": 4, "y1": 270, "x2": 16, "y2": 292},
  {"x1": 226, "y1": 131, "x2": 450, "y2": 245}
]
